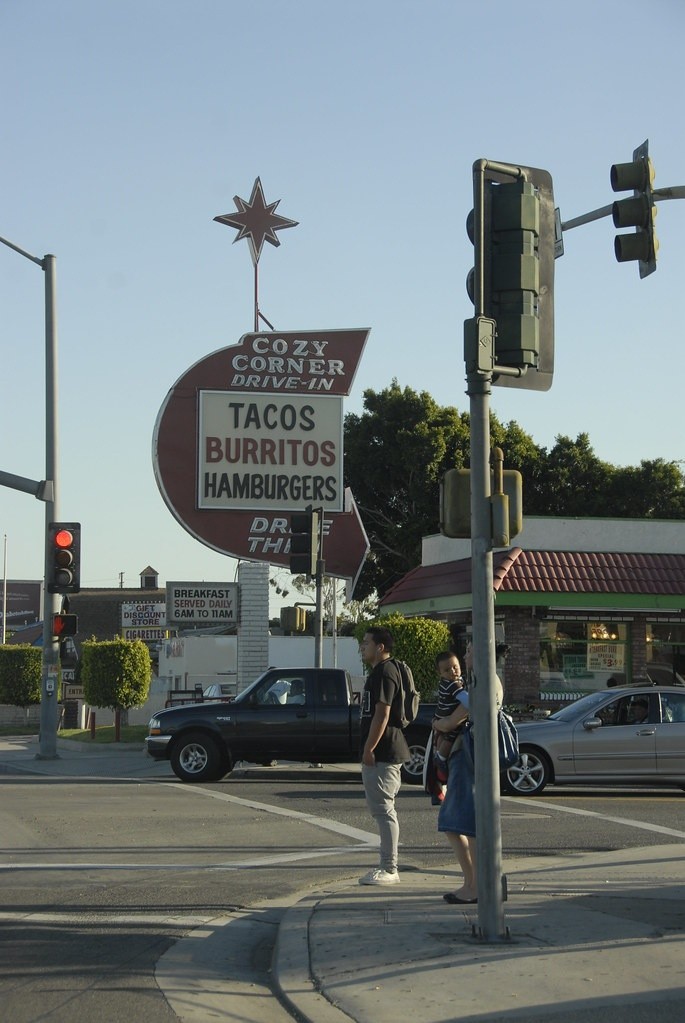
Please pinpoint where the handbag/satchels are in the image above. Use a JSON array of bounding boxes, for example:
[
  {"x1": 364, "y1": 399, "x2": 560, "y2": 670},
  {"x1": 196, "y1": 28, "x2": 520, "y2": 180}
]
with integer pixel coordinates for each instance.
[{"x1": 462, "y1": 709, "x2": 519, "y2": 773}]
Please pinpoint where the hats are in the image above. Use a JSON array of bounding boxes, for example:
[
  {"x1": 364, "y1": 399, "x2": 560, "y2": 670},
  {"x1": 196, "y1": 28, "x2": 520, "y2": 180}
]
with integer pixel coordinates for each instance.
[{"x1": 631, "y1": 698, "x2": 648, "y2": 709}]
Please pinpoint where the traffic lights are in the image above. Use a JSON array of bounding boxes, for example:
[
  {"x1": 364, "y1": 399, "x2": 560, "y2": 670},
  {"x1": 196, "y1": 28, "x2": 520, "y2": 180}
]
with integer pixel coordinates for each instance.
[
  {"x1": 47, "y1": 522, "x2": 82, "y2": 593},
  {"x1": 611, "y1": 139, "x2": 659, "y2": 278},
  {"x1": 50, "y1": 614, "x2": 79, "y2": 637},
  {"x1": 464, "y1": 160, "x2": 557, "y2": 390},
  {"x1": 291, "y1": 503, "x2": 317, "y2": 576}
]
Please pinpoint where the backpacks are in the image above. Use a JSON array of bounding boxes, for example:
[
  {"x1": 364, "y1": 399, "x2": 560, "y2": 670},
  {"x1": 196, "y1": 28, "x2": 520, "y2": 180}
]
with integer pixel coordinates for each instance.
[{"x1": 391, "y1": 657, "x2": 420, "y2": 728}]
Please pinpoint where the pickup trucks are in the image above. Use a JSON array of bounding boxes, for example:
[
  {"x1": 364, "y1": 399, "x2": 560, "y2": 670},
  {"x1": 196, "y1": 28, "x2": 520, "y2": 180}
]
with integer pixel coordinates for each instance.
[{"x1": 141, "y1": 668, "x2": 441, "y2": 782}]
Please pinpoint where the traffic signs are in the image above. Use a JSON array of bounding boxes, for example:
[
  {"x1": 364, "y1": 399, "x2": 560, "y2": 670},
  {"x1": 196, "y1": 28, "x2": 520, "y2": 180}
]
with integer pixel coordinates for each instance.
[{"x1": 149, "y1": 327, "x2": 371, "y2": 602}]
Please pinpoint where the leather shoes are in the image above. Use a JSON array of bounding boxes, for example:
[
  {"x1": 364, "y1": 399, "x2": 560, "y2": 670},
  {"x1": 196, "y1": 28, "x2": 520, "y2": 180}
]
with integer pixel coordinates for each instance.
[{"x1": 443, "y1": 892, "x2": 478, "y2": 904}]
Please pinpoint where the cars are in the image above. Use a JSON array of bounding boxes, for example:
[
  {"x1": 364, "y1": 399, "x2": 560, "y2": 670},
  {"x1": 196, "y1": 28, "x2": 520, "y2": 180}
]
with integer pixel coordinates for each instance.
[
  {"x1": 499, "y1": 681, "x2": 684, "y2": 795},
  {"x1": 203, "y1": 681, "x2": 235, "y2": 703}
]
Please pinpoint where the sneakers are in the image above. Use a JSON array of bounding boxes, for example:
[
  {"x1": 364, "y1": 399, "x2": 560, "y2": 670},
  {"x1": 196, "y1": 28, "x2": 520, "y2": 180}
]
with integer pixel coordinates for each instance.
[{"x1": 358, "y1": 868, "x2": 400, "y2": 885}]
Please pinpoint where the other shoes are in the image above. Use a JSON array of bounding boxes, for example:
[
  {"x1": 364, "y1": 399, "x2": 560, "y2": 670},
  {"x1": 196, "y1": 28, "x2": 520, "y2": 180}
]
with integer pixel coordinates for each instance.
[{"x1": 434, "y1": 755, "x2": 449, "y2": 783}]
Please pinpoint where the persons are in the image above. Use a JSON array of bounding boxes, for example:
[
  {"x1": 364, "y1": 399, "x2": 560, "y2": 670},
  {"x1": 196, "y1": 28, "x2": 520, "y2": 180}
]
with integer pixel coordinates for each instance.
[
  {"x1": 358, "y1": 628, "x2": 410, "y2": 885},
  {"x1": 429, "y1": 651, "x2": 470, "y2": 805},
  {"x1": 431, "y1": 641, "x2": 508, "y2": 905},
  {"x1": 267, "y1": 679, "x2": 306, "y2": 705}
]
